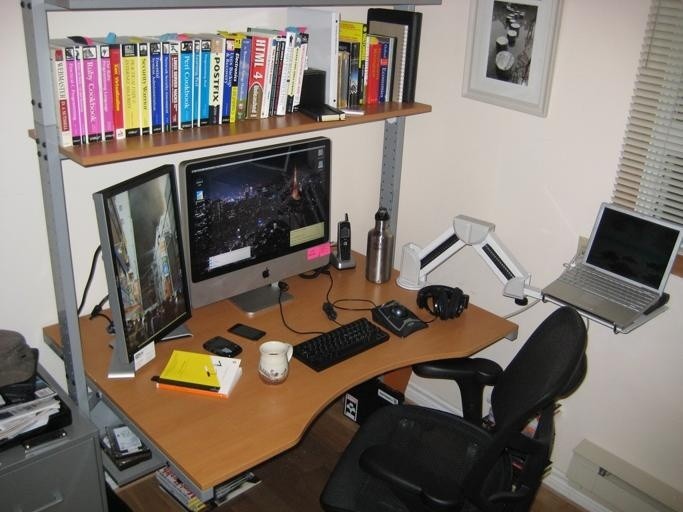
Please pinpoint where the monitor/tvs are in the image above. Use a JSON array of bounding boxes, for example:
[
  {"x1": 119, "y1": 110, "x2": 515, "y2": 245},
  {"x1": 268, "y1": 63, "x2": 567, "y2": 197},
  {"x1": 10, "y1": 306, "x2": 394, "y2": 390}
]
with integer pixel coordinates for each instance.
[{"x1": 181, "y1": 136, "x2": 333, "y2": 309}]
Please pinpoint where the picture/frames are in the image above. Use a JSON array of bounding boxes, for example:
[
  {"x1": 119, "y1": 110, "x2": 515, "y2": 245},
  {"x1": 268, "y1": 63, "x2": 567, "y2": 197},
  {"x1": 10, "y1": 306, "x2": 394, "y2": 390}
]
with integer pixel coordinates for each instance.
[{"x1": 459, "y1": 0, "x2": 564, "y2": 118}]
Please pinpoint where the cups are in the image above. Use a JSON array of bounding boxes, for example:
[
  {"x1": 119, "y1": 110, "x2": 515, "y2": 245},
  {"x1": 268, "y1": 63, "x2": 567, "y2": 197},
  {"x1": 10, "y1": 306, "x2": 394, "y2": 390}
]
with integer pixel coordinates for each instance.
[{"x1": 257, "y1": 341, "x2": 293, "y2": 384}]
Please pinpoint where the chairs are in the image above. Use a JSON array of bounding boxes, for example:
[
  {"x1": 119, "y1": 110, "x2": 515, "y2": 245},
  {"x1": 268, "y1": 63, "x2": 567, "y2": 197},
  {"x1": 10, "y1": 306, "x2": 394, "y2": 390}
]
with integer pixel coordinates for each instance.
[{"x1": 320, "y1": 307, "x2": 589, "y2": 510}]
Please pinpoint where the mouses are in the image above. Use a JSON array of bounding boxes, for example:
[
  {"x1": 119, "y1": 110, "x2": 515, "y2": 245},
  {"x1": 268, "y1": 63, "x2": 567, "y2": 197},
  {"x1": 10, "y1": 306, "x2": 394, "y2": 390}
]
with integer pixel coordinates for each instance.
[{"x1": 374, "y1": 300, "x2": 415, "y2": 333}]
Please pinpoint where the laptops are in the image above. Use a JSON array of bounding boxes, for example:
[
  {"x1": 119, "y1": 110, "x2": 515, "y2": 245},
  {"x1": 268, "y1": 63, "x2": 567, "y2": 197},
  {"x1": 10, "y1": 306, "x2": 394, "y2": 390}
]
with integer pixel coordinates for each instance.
[{"x1": 539, "y1": 200, "x2": 681, "y2": 329}]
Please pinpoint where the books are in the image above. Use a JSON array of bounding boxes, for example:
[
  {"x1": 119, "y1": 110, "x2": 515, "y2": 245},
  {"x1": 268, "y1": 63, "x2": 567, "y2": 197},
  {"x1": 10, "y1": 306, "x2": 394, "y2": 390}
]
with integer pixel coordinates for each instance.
[
  {"x1": 0, "y1": 369, "x2": 72, "y2": 452},
  {"x1": 154, "y1": 466, "x2": 262, "y2": 512},
  {"x1": 156, "y1": 350, "x2": 243, "y2": 398}
]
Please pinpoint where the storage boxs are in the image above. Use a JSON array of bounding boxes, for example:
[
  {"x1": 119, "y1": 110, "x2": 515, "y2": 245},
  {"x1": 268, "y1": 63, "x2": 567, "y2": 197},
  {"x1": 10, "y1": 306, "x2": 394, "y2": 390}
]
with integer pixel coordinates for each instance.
[{"x1": 0, "y1": 361, "x2": 110, "y2": 511}]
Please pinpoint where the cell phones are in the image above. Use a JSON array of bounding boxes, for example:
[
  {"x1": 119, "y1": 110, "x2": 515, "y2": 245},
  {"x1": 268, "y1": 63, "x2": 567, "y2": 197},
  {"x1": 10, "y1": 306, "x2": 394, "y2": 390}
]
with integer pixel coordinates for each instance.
[
  {"x1": 228, "y1": 323, "x2": 265, "y2": 340},
  {"x1": 203, "y1": 336, "x2": 242, "y2": 358}
]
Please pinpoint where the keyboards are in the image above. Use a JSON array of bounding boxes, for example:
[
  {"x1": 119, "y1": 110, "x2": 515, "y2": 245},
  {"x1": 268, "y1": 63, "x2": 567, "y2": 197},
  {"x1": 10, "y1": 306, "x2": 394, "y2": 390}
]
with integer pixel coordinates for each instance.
[{"x1": 293, "y1": 316, "x2": 388, "y2": 371}]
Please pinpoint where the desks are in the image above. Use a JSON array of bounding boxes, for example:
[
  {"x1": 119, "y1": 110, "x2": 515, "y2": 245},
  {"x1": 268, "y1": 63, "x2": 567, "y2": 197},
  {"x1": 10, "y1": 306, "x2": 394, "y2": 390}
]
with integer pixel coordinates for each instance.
[{"x1": 43, "y1": 242, "x2": 518, "y2": 480}]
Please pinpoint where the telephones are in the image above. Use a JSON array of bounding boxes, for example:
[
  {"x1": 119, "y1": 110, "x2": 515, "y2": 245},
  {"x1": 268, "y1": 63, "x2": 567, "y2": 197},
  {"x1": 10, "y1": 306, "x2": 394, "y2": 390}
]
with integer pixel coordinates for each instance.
[{"x1": 332, "y1": 213, "x2": 357, "y2": 271}]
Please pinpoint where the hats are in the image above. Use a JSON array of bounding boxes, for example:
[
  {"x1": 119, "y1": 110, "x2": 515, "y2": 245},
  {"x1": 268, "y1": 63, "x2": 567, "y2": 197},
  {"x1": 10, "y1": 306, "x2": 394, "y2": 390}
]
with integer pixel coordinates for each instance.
[{"x1": 0, "y1": 328, "x2": 36, "y2": 387}]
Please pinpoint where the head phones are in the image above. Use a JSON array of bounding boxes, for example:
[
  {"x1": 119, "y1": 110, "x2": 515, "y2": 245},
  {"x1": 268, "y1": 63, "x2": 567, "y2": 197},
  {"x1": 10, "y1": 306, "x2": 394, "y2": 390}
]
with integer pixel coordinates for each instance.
[{"x1": 413, "y1": 283, "x2": 472, "y2": 318}]
